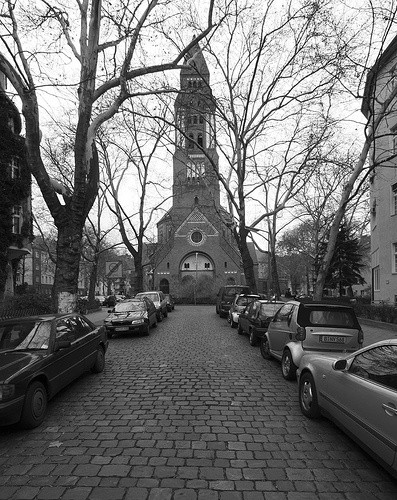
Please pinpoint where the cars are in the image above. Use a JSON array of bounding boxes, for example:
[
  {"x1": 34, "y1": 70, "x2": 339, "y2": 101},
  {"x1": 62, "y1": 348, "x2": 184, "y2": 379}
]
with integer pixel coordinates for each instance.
[
  {"x1": 259, "y1": 299, "x2": 365, "y2": 380},
  {"x1": 237, "y1": 300, "x2": 287, "y2": 346},
  {"x1": 0, "y1": 313, "x2": 109, "y2": 429},
  {"x1": 104, "y1": 296, "x2": 157, "y2": 339},
  {"x1": 297, "y1": 337, "x2": 397, "y2": 481},
  {"x1": 135, "y1": 291, "x2": 175, "y2": 322},
  {"x1": 227, "y1": 292, "x2": 261, "y2": 328}
]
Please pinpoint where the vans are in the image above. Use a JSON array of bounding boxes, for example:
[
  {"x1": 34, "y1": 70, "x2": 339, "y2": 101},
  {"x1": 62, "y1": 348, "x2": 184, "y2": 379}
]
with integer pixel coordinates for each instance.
[{"x1": 216, "y1": 284, "x2": 251, "y2": 317}]
